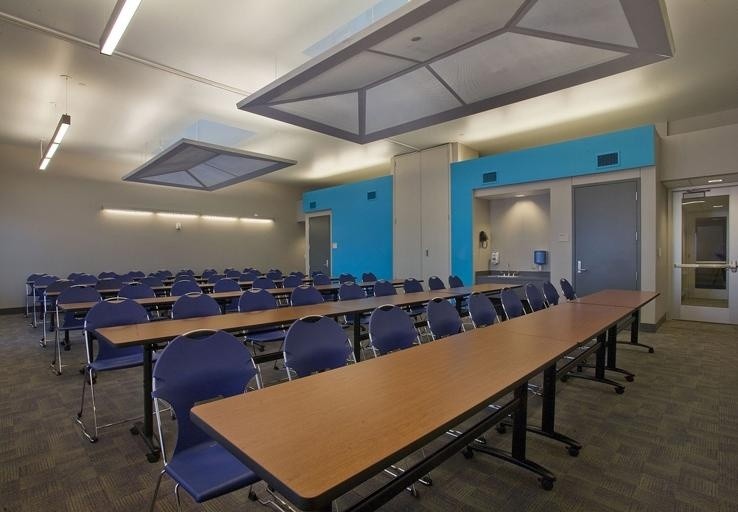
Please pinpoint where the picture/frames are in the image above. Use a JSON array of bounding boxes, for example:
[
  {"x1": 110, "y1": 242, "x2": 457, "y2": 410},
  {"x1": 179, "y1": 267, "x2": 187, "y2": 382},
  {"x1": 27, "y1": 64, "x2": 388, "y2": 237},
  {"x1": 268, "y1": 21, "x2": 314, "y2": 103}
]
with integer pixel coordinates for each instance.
[
  {"x1": 568, "y1": 288, "x2": 660, "y2": 381},
  {"x1": 485, "y1": 302, "x2": 635, "y2": 456}
]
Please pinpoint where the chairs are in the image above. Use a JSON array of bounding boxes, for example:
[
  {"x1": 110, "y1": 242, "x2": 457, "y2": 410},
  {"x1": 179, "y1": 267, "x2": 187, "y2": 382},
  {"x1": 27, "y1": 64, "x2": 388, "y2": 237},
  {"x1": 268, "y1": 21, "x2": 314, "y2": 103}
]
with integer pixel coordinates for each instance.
[{"x1": 26, "y1": 269, "x2": 577, "y2": 511}]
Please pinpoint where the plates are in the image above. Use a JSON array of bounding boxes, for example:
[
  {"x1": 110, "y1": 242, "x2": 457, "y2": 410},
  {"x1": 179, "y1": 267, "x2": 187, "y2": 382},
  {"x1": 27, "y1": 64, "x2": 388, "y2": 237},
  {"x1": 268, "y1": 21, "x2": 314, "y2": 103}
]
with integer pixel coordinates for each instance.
[{"x1": 393, "y1": 143, "x2": 479, "y2": 288}]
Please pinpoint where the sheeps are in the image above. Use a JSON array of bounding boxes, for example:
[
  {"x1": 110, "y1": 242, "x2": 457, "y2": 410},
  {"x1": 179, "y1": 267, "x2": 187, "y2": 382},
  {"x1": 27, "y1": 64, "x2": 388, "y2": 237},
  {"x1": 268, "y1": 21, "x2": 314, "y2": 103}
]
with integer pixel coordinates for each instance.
[{"x1": 506, "y1": 271, "x2": 511, "y2": 276}]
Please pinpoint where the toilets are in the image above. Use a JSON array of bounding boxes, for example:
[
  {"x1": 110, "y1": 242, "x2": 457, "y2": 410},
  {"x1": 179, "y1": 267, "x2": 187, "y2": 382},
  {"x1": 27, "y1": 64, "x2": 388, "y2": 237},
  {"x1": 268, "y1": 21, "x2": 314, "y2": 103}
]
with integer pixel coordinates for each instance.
[{"x1": 480, "y1": 231, "x2": 488, "y2": 241}]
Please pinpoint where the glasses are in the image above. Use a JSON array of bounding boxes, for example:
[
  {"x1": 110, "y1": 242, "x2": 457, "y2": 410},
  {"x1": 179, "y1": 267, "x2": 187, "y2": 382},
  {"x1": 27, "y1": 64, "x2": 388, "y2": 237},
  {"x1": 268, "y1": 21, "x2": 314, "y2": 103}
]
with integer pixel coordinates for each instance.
[
  {"x1": 38, "y1": 75, "x2": 71, "y2": 170},
  {"x1": 237, "y1": 1, "x2": 674, "y2": 144},
  {"x1": 121, "y1": 123, "x2": 297, "y2": 191},
  {"x1": 100, "y1": 0, "x2": 141, "y2": 56}
]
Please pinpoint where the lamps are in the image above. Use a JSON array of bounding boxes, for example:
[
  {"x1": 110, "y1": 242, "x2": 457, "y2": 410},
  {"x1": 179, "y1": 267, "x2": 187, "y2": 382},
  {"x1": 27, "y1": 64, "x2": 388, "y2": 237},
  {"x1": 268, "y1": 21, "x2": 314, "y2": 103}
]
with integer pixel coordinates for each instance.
[
  {"x1": 237, "y1": 1, "x2": 674, "y2": 144},
  {"x1": 38, "y1": 75, "x2": 71, "y2": 170},
  {"x1": 121, "y1": 123, "x2": 297, "y2": 191},
  {"x1": 100, "y1": 0, "x2": 141, "y2": 56}
]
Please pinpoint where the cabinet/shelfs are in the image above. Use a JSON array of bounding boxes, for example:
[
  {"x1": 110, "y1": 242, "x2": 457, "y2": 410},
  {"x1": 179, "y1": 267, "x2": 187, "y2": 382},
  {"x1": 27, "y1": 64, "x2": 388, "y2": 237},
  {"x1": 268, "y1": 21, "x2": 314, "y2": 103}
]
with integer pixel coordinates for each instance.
[{"x1": 393, "y1": 143, "x2": 479, "y2": 288}]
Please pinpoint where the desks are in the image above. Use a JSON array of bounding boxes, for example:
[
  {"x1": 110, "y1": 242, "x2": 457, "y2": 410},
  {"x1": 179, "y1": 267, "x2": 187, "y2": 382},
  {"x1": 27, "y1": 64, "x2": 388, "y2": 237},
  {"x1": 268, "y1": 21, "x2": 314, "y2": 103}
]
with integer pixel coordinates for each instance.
[
  {"x1": 568, "y1": 288, "x2": 660, "y2": 381},
  {"x1": 485, "y1": 302, "x2": 635, "y2": 456}
]
